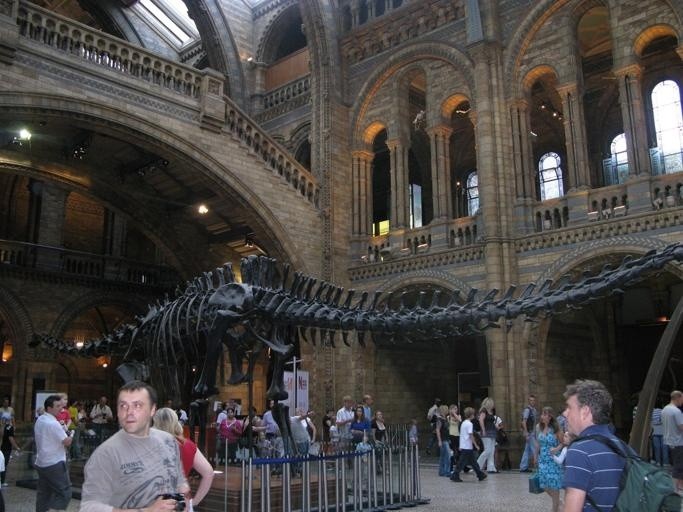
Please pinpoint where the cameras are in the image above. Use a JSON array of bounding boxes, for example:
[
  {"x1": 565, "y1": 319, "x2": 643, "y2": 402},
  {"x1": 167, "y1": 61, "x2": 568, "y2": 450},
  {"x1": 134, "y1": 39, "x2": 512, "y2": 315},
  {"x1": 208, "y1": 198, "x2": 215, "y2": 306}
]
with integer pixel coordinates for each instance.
[{"x1": 156, "y1": 492, "x2": 186, "y2": 512}]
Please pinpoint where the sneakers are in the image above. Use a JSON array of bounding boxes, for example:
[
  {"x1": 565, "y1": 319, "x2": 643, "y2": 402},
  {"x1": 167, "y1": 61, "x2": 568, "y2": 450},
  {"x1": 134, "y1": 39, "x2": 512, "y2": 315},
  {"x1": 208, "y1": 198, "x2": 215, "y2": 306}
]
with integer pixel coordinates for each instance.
[
  {"x1": 450, "y1": 477, "x2": 463, "y2": 482},
  {"x1": 520, "y1": 469, "x2": 532, "y2": 472},
  {"x1": 487, "y1": 470, "x2": 500, "y2": 474},
  {"x1": 479, "y1": 474, "x2": 486, "y2": 481}
]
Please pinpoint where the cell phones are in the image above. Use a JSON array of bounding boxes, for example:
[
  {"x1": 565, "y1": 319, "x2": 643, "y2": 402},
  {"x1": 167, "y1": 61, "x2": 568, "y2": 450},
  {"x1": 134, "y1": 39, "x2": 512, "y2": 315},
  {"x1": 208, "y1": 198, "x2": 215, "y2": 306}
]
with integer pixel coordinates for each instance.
[{"x1": 103, "y1": 413, "x2": 106, "y2": 420}]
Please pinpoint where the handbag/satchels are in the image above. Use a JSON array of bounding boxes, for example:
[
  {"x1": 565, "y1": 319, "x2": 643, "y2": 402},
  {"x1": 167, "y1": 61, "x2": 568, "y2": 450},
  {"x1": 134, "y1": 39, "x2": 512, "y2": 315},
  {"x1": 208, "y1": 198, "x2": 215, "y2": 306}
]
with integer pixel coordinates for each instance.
[{"x1": 528, "y1": 463, "x2": 544, "y2": 494}]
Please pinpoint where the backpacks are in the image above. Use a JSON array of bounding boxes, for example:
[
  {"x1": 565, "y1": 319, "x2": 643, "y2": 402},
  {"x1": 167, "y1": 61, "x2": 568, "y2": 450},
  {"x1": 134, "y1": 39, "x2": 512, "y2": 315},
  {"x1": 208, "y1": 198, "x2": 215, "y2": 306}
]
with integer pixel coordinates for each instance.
[{"x1": 571, "y1": 435, "x2": 683, "y2": 512}]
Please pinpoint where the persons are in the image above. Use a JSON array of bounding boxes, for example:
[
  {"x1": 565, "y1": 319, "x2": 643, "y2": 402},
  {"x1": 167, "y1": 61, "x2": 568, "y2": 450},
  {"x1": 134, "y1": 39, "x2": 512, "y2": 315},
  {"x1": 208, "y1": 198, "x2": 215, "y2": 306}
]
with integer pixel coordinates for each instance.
[
  {"x1": 1, "y1": 413, "x2": 20, "y2": 486},
  {"x1": 436, "y1": 405, "x2": 450, "y2": 477},
  {"x1": 89, "y1": 396, "x2": 113, "y2": 433},
  {"x1": 362, "y1": 395, "x2": 373, "y2": 421},
  {"x1": 67, "y1": 400, "x2": 78, "y2": 449},
  {"x1": 350, "y1": 406, "x2": 371, "y2": 450},
  {"x1": 80, "y1": 381, "x2": 187, "y2": 512},
  {"x1": 552, "y1": 432, "x2": 570, "y2": 465},
  {"x1": 562, "y1": 379, "x2": 640, "y2": 512},
  {"x1": 153, "y1": 410, "x2": 214, "y2": 511},
  {"x1": 57, "y1": 394, "x2": 72, "y2": 432},
  {"x1": 492, "y1": 408, "x2": 504, "y2": 469},
  {"x1": 536, "y1": 408, "x2": 563, "y2": 510},
  {"x1": 371, "y1": 411, "x2": 388, "y2": 475},
  {"x1": 322, "y1": 407, "x2": 336, "y2": 443},
  {"x1": 449, "y1": 404, "x2": 461, "y2": 462},
  {"x1": 216, "y1": 402, "x2": 230, "y2": 434},
  {"x1": 631, "y1": 394, "x2": 642, "y2": 429},
  {"x1": 425, "y1": 399, "x2": 442, "y2": 456},
  {"x1": 35, "y1": 395, "x2": 72, "y2": 512},
  {"x1": 478, "y1": 397, "x2": 497, "y2": 474},
  {"x1": 661, "y1": 390, "x2": 683, "y2": 489},
  {"x1": 0, "y1": 400, "x2": 16, "y2": 430},
  {"x1": 241, "y1": 406, "x2": 261, "y2": 432},
  {"x1": 239, "y1": 417, "x2": 268, "y2": 449},
  {"x1": 651, "y1": 399, "x2": 664, "y2": 463},
  {"x1": 336, "y1": 396, "x2": 355, "y2": 443},
  {"x1": 263, "y1": 400, "x2": 279, "y2": 439},
  {"x1": 450, "y1": 408, "x2": 487, "y2": 482},
  {"x1": 520, "y1": 395, "x2": 536, "y2": 472},
  {"x1": 290, "y1": 410, "x2": 315, "y2": 455},
  {"x1": 295, "y1": 407, "x2": 315, "y2": 443},
  {"x1": 407, "y1": 419, "x2": 421, "y2": 462},
  {"x1": 220, "y1": 408, "x2": 242, "y2": 459}
]
